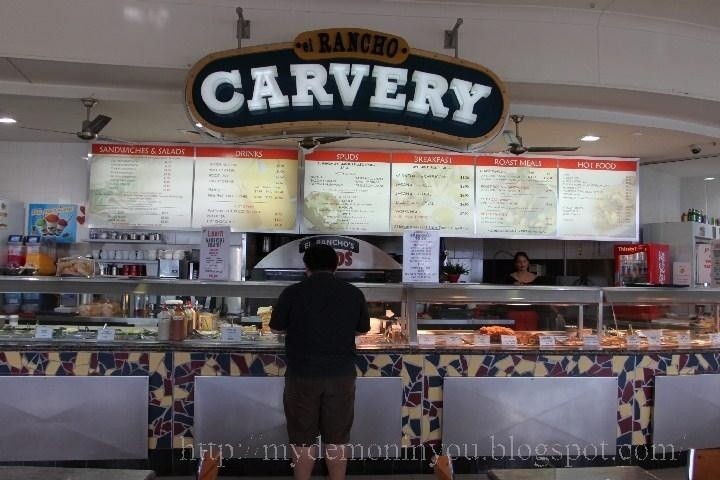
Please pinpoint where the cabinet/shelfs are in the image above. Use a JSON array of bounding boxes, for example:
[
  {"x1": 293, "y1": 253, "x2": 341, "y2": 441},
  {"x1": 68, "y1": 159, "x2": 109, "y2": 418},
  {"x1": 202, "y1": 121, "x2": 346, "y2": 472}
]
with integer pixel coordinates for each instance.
[{"x1": 80, "y1": 238, "x2": 166, "y2": 265}]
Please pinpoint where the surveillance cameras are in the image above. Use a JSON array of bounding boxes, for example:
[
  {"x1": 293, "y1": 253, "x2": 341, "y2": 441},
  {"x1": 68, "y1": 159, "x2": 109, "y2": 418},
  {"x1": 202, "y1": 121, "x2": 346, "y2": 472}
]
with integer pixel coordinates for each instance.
[{"x1": 689, "y1": 145, "x2": 703, "y2": 154}]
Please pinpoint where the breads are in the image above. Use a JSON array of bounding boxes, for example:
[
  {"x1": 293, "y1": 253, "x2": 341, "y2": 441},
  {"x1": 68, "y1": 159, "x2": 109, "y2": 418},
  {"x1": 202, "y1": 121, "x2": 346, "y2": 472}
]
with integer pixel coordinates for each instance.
[{"x1": 197, "y1": 306, "x2": 714, "y2": 350}]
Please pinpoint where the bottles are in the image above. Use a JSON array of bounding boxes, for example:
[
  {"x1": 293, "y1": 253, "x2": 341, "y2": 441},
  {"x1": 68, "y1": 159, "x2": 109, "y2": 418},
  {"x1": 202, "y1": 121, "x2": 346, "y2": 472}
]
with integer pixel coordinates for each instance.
[
  {"x1": 228, "y1": 244, "x2": 242, "y2": 282},
  {"x1": 680, "y1": 207, "x2": 720, "y2": 226},
  {"x1": 620, "y1": 251, "x2": 647, "y2": 282},
  {"x1": 156, "y1": 301, "x2": 199, "y2": 343}
]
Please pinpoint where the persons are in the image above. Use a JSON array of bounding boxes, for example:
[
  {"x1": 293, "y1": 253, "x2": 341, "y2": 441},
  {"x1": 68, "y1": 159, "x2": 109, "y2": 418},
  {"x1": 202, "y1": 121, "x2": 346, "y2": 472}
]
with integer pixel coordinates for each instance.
[
  {"x1": 502, "y1": 251, "x2": 539, "y2": 330},
  {"x1": 267, "y1": 243, "x2": 372, "y2": 480}
]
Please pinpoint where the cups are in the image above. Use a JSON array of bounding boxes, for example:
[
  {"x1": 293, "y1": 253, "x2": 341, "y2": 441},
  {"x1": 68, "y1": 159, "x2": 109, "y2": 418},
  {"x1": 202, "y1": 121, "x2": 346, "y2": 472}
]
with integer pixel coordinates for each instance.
[
  {"x1": 120, "y1": 293, "x2": 131, "y2": 314},
  {"x1": 134, "y1": 293, "x2": 146, "y2": 312},
  {"x1": 82, "y1": 229, "x2": 186, "y2": 276},
  {"x1": 187, "y1": 259, "x2": 200, "y2": 281},
  {"x1": 36, "y1": 220, "x2": 66, "y2": 236}
]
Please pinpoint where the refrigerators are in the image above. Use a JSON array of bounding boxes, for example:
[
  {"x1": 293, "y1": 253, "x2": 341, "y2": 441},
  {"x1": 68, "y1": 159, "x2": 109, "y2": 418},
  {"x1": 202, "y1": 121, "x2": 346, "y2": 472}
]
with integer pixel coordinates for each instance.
[{"x1": 613, "y1": 243, "x2": 671, "y2": 320}]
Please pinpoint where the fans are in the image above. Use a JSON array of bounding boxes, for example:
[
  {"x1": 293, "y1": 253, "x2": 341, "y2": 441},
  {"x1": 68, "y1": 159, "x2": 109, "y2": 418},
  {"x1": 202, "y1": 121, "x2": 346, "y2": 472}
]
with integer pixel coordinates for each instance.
[
  {"x1": 297, "y1": 136, "x2": 352, "y2": 150},
  {"x1": 500, "y1": 115, "x2": 579, "y2": 155},
  {"x1": 19, "y1": 97, "x2": 131, "y2": 143}
]
